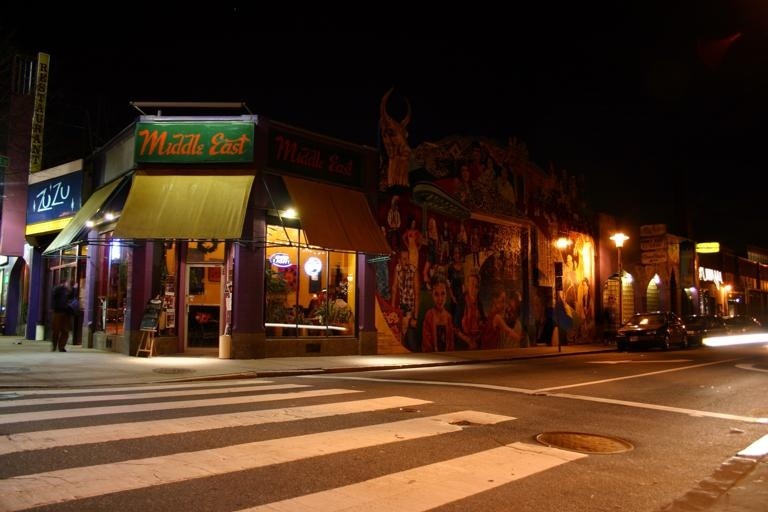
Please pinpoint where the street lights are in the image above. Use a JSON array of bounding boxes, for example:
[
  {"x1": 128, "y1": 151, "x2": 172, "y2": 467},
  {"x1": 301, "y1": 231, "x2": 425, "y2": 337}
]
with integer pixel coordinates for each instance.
[{"x1": 609, "y1": 233, "x2": 630, "y2": 326}]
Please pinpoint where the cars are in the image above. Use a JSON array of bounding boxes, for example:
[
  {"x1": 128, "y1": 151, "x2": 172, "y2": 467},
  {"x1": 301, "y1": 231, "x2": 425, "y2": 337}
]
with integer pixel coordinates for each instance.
[{"x1": 616, "y1": 311, "x2": 768, "y2": 351}]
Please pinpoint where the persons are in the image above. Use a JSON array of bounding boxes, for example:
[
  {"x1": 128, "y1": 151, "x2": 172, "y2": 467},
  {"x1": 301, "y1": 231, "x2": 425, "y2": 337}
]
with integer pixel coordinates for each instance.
[
  {"x1": 386, "y1": 195, "x2": 594, "y2": 352},
  {"x1": 51, "y1": 279, "x2": 78, "y2": 352}
]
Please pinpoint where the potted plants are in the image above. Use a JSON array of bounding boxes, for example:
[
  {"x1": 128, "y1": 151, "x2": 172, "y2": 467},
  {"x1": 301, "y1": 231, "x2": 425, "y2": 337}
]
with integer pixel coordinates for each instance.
[{"x1": 266, "y1": 300, "x2": 354, "y2": 336}]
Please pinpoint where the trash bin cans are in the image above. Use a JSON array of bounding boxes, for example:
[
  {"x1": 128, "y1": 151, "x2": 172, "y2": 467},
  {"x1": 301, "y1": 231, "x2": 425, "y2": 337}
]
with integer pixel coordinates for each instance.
[
  {"x1": 35, "y1": 322, "x2": 45, "y2": 341},
  {"x1": 219, "y1": 335, "x2": 231, "y2": 359}
]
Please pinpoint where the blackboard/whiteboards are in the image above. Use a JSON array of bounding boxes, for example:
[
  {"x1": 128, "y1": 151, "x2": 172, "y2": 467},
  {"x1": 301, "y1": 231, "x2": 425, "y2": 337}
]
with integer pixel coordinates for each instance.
[
  {"x1": 189, "y1": 267, "x2": 205, "y2": 296},
  {"x1": 140, "y1": 299, "x2": 163, "y2": 330}
]
